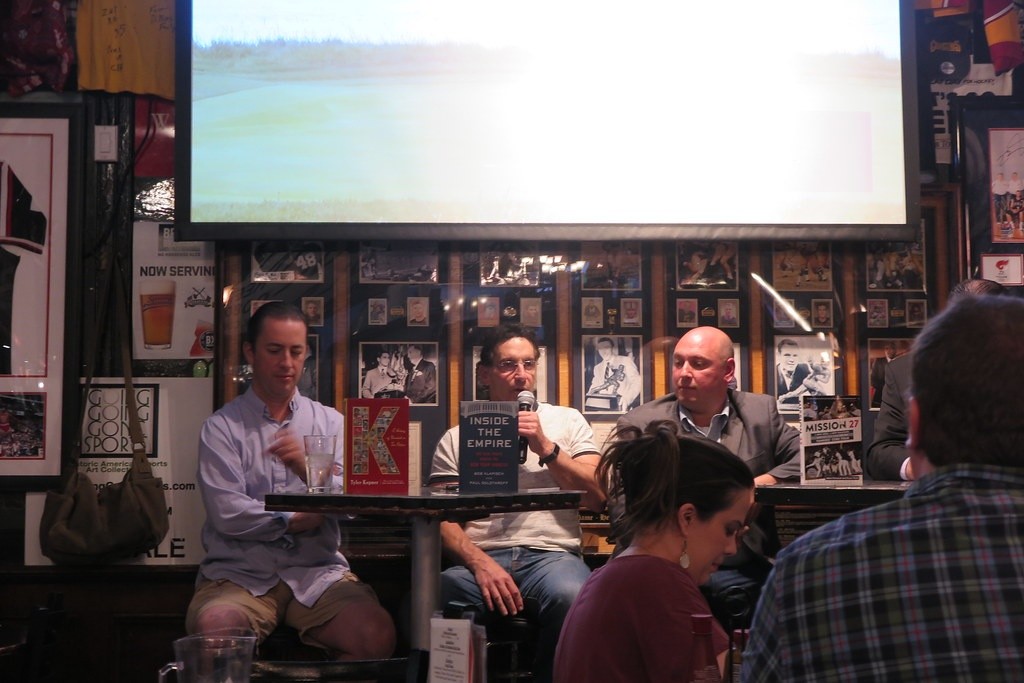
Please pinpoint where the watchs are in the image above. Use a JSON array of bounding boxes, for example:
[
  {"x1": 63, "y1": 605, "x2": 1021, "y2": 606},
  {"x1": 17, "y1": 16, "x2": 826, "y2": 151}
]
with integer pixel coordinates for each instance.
[{"x1": 538, "y1": 442, "x2": 560, "y2": 467}]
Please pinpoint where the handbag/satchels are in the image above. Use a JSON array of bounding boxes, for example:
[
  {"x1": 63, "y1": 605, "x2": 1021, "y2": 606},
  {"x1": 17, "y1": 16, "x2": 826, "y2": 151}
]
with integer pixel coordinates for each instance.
[{"x1": 38, "y1": 454, "x2": 170, "y2": 569}]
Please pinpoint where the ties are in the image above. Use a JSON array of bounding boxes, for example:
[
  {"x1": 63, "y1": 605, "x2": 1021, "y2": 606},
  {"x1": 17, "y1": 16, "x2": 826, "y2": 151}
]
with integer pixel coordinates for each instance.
[
  {"x1": 380, "y1": 369, "x2": 385, "y2": 379},
  {"x1": 604, "y1": 362, "x2": 610, "y2": 390}
]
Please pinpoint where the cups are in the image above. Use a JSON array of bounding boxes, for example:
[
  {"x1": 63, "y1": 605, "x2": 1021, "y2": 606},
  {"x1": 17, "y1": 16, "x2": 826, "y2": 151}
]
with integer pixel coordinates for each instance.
[
  {"x1": 303, "y1": 432, "x2": 338, "y2": 494},
  {"x1": 139, "y1": 275, "x2": 176, "y2": 349}
]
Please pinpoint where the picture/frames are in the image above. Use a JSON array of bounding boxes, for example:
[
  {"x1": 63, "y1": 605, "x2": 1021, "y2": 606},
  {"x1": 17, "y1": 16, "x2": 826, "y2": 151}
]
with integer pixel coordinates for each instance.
[{"x1": 0, "y1": 100, "x2": 87, "y2": 490}]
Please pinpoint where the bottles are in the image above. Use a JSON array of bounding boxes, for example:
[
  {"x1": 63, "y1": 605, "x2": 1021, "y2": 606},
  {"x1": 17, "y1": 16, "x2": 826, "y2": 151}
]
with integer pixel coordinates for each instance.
[{"x1": 676, "y1": 613, "x2": 751, "y2": 683}]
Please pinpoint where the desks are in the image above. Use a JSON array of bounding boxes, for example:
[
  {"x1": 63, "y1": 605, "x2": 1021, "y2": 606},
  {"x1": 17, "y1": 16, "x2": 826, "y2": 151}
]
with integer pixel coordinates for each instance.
[{"x1": 264, "y1": 489, "x2": 586, "y2": 654}]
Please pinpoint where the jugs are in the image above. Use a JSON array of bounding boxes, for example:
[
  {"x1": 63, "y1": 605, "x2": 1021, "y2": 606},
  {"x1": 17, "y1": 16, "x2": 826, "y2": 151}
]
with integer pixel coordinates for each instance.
[{"x1": 158, "y1": 627, "x2": 258, "y2": 683}]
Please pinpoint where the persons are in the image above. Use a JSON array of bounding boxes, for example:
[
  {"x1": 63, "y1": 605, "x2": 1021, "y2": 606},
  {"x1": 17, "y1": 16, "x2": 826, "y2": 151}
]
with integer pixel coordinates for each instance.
[
  {"x1": 806, "y1": 447, "x2": 864, "y2": 477},
  {"x1": 611, "y1": 324, "x2": 801, "y2": 645},
  {"x1": 551, "y1": 421, "x2": 756, "y2": 683},
  {"x1": 242, "y1": 244, "x2": 930, "y2": 417},
  {"x1": 865, "y1": 278, "x2": 1009, "y2": 482},
  {"x1": 431, "y1": 321, "x2": 609, "y2": 683},
  {"x1": 184, "y1": 302, "x2": 397, "y2": 683},
  {"x1": 739, "y1": 293, "x2": 1024, "y2": 683}
]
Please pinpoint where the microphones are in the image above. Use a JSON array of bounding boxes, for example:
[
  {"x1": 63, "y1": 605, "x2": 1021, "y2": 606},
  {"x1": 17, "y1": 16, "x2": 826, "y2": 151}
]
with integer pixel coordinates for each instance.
[{"x1": 518, "y1": 390, "x2": 535, "y2": 464}]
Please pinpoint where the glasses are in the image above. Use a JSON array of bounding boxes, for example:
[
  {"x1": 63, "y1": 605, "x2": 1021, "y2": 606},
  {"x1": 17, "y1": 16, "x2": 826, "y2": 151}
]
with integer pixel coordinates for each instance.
[{"x1": 488, "y1": 360, "x2": 538, "y2": 372}]
